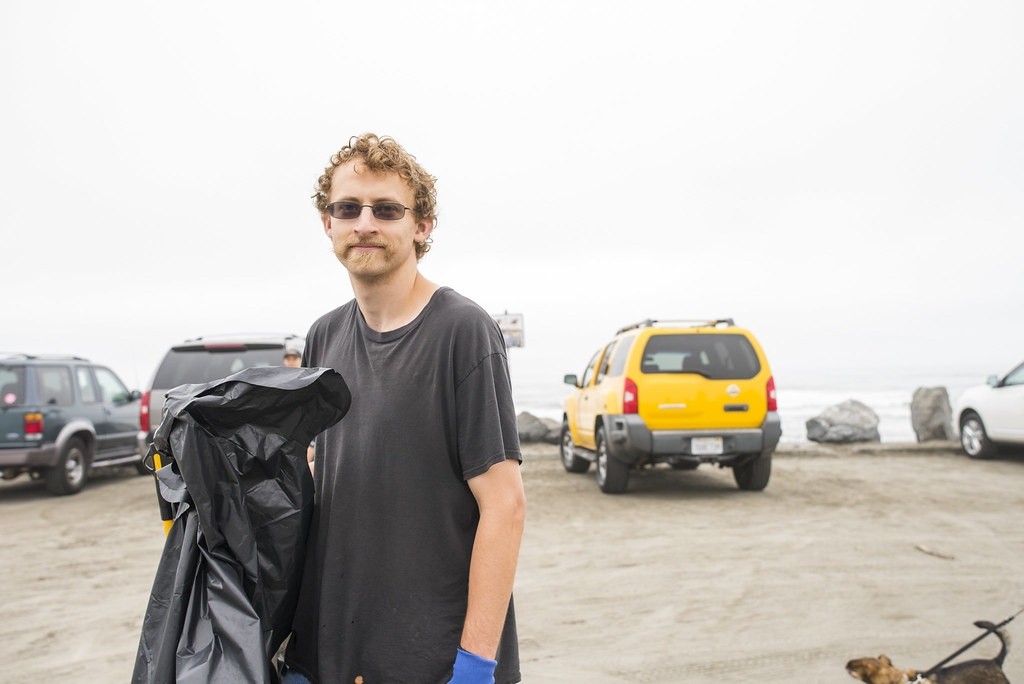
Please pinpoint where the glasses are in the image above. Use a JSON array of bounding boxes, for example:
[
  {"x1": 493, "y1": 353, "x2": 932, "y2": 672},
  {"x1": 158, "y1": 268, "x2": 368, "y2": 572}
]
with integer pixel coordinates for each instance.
[{"x1": 323, "y1": 201, "x2": 414, "y2": 220}]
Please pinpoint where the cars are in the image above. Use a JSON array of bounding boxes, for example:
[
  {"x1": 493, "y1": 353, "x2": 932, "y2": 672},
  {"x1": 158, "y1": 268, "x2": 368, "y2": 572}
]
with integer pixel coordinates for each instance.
[{"x1": 948, "y1": 362, "x2": 1024, "y2": 461}]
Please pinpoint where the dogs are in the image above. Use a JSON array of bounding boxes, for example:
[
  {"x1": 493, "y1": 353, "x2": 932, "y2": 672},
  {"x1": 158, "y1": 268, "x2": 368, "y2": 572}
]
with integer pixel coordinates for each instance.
[{"x1": 845, "y1": 619, "x2": 1011, "y2": 684}]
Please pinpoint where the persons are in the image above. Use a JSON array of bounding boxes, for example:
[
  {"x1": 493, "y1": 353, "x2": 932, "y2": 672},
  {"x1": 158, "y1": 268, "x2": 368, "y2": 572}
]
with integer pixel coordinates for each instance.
[
  {"x1": 283, "y1": 349, "x2": 302, "y2": 368},
  {"x1": 301, "y1": 134, "x2": 524, "y2": 684}
]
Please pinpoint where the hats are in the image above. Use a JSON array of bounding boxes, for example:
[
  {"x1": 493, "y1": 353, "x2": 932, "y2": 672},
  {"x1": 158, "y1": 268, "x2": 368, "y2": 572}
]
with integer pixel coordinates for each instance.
[{"x1": 284, "y1": 348, "x2": 301, "y2": 357}]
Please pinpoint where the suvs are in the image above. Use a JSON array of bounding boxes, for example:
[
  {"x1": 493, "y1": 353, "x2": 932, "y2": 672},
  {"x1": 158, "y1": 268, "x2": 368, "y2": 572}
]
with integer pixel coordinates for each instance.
[
  {"x1": 560, "y1": 317, "x2": 783, "y2": 497},
  {"x1": 0, "y1": 354, "x2": 157, "y2": 499},
  {"x1": 138, "y1": 333, "x2": 305, "y2": 474}
]
